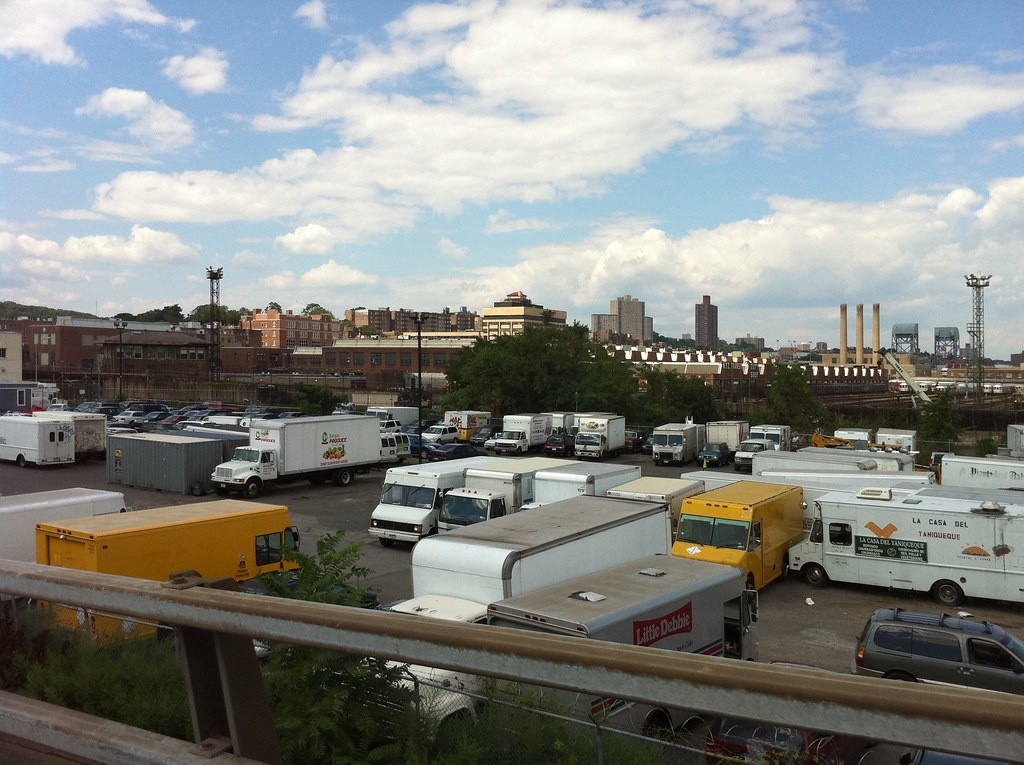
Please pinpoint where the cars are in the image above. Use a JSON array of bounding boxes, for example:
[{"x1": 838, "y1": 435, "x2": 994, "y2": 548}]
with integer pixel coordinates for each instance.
[
  {"x1": 484, "y1": 431, "x2": 503, "y2": 451},
  {"x1": 430, "y1": 442, "x2": 490, "y2": 464},
  {"x1": 45, "y1": 398, "x2": 307, "y2": 432}
]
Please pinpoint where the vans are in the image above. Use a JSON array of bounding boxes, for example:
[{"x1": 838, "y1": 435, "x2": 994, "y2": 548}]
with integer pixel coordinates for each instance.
[
  {"x1": 734, "y1": 438, "x2": 775, "y2": 472},
  {"x1": 470, "y1": 424, "x2": 503, "y2": 447}
]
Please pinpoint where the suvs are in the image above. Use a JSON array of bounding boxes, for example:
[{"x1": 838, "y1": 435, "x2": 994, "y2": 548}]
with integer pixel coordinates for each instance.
[
  {"x1": 332, "y1": 402, "x2": 358, "y2": 417},
  {"x1": 375, "y1": 420, "x2": 458, "y2": 464},
  {"x1": 697, "y1": 441, "x2": 731, "y2": 469},
  {"x1": 422, "y1": 425, "x2": 459, "y2": 446},
  {"x1": 543, "y1": 433, "x2": 575, "y2": 458},
  {"x1": 853, "y1": 606, "x2": 1024, "y2": 696}
]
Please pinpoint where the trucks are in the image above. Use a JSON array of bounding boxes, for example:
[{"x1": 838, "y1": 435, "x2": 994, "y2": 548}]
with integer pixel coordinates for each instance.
[
  {"x1": 787, "y1": 488, "x2": 1024, "y2": 609},
  {"x1": 4, "y1": 410, "x2": 107, "y2": 462},
  {"x1": 347, "y1": 495, "x2": 674, "y2": 748},
  {"x1": 0, "y1": 416, "x2": 76, "y2": 468},
  {"x1": 369, "y1": 405, "x2": 420, "y2": 426},
  {"x1": 542, "y1": 411, "x2": 580, "y2": 437},
  {"x1": 517, "y1": 462, "x2": 640, "y2": 532},
  {"x1": 437, "y1": 457, "x2": 582, "y2": 533},
  {"x1": 670, "y1": 479, "x2": 806, "y2": 594},
  {"x1": 607, "y1": 476, "x2": 706, "y2": 538},
  {"x1": 443, "y1": 410, "x2": 492, "y2": 442},
  {"x1": 211, "y1": 414, "x2": 384, "y2": 500},
  {"x1": 494, "y1": 413, "x2": 554, "y2": 457},
  {"x1": 37, "y1": 497, "x2": 303, "y2": 660},
  {"x1": 0, "y1": 487, "x2": 133, "y2": 614},
  {"x1": 486, "y1": 553, "x2": 759, "y2": 745},
  {"x1": 367, "y1": 455, "x2": 517, "y2": 548},
  {"x1": 570, "y1": 411, "x2": 1024, "y2": 551}
]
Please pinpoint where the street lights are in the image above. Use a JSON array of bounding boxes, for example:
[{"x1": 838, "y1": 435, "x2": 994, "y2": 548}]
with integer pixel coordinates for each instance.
[
  {"x1": 113, "y1": 320, "x2": 128, "y2": 402},
  {"x1": 410, "y1": 310, "x2": 427, "y2": 462}
]
